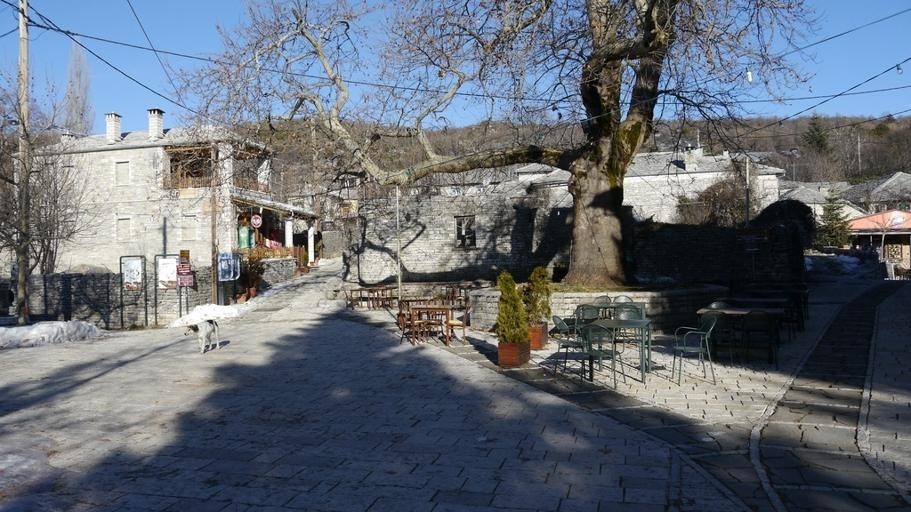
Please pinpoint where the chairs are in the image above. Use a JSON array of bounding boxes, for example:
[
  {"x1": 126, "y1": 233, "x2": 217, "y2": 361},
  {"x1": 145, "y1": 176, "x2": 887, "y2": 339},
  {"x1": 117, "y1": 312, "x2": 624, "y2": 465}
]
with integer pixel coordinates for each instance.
[
  {"x1": 554, "y1": 296, "x2": 718, "y2": 388},
  {"x1": 342, "y1": 284, "x2": 471, "y2": 346}
]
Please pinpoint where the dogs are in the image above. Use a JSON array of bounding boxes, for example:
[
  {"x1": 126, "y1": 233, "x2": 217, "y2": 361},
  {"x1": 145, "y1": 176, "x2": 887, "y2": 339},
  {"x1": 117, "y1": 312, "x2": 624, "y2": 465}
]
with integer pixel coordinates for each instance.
[{"x1": 183, "y1": 319, "x2": 221, "y2": 354}]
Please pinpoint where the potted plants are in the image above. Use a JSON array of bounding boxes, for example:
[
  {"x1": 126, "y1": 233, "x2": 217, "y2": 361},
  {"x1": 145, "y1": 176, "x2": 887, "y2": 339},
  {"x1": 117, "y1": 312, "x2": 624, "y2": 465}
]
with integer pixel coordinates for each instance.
[
  {"x1": 497, "y1": 263, "x2": 550, "y2": 368},
  {"x1": 233, "y1": 260, "x2": 265, "y2": 303}
]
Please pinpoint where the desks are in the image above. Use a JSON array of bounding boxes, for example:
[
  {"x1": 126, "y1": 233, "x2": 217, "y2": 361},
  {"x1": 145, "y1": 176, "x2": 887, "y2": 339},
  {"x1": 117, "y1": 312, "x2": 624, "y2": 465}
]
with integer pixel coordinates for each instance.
[{"x1": 697, "y1": 281, "x2": 813, "y2": 369}]
